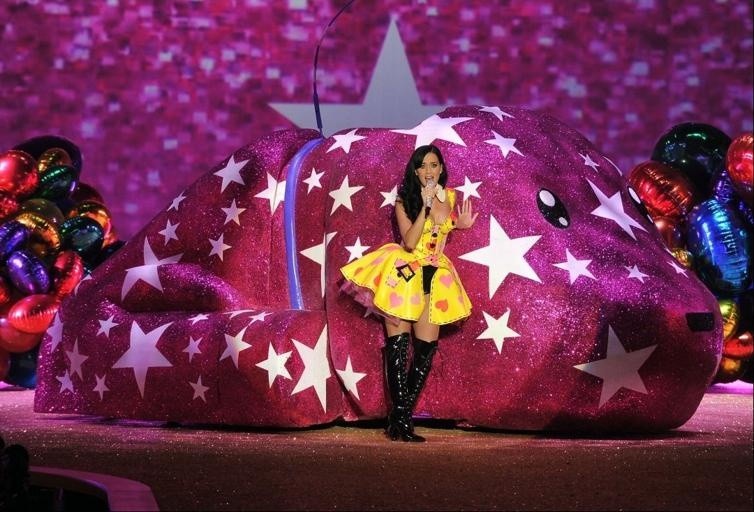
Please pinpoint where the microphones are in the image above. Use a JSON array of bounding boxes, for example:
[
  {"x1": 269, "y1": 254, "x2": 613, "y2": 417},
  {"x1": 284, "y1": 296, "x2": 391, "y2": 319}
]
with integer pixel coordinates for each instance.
[{"x1": 426, "y1": 180, "x2": 434, "y2": 215}]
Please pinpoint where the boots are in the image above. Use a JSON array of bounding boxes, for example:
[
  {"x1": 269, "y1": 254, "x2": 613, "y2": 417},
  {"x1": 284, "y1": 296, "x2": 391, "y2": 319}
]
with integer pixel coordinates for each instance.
[{"x1": 380, "y1": 330, "x2": 440, "y2": 443}]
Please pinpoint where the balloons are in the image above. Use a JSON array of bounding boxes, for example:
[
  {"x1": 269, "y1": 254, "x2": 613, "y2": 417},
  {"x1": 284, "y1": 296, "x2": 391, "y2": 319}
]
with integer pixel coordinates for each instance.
[
  {"x1": 0, "y1": 134, "x2": 124, "y2": 388},
  {"x1": 629, "y1": 119, "x2": 753, "y2": 383}
]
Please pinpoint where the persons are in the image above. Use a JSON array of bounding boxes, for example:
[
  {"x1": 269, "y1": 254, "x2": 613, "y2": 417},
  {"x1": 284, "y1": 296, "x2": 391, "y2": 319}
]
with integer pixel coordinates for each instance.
[{"x1": 339, "y1": 143, "x2": 479, "y2": 442}]
